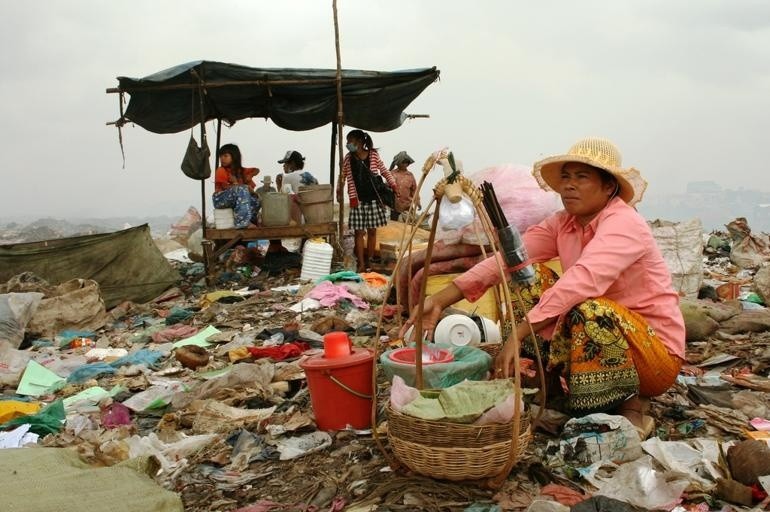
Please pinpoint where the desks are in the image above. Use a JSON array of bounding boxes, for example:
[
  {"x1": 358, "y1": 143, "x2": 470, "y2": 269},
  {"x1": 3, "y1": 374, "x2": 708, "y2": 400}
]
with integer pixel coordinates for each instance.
[{"x1": 200, "y1": 223, "x2": 339, "y2": 287}]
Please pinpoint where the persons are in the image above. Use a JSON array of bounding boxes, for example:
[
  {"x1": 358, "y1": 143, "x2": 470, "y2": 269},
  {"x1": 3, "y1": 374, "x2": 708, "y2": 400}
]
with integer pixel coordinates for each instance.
[
  {"x1": 386, "y1": 151, "x2": 422, "y2": 220},
  {"x1": 255, "y1": 175, "x2": 276, "y2": 192},
  {"x1": 212, "y1": 143, "x2": 262, "y2": 228},
  {"x1": 336, "y1": 129, "x2": 403, "y2": 272},
  {"x1": 276, "y1": 149, "x2": 319, "y2": 224},
  {"x1": 397, "y1": 136, "x2": 688, "y2": 442}
]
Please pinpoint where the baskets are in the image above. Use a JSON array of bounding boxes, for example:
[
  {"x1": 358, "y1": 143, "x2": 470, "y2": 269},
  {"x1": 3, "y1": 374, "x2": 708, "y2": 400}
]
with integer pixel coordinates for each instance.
[{"x1": 385, "y1": 390, "x2": 533, "y2": 481}]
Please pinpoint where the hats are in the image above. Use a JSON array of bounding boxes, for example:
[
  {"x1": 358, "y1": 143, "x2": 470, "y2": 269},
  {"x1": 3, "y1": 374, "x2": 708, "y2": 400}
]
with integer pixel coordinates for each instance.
[
  {"x1": 531, "y1": 137, "x2": 647, "y2": 208},
  {"x1": 278, "y1": 151, "x2": 305, "y2": 163},
  {"x1": 261, "y1": 176, "x2": 274, "y2": 184},
  {"x1": 394, "y1": 151, "x2": 415, "y2": 165}
]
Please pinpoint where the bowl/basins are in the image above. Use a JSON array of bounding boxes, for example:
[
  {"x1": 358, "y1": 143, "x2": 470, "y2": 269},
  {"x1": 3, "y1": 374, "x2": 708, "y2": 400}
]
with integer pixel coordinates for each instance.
[
  {"x1": 434, "y1": 314, "x2": 481, "y2": 347},
  {"x1": 481, "y1": 316, "x2": 499, "y2": 343}
]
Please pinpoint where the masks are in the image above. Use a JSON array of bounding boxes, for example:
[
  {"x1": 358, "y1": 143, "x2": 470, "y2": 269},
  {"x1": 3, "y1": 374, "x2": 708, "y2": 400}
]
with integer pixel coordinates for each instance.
[{"x1": 346, "y1": 143, "x2": 359, "y2": 152}]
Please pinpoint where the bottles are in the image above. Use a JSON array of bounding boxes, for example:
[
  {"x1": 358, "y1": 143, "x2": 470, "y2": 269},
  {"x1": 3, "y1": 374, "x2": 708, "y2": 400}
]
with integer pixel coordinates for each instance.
[{"x1": 493, "y1": 221, "x2": 535, "y2": 288}]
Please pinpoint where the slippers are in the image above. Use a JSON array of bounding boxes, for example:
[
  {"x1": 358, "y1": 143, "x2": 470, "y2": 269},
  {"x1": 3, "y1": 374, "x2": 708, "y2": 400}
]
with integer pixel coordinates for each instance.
[{"x1": 635, "y1": 415, "x2": 655, "y2": 441}]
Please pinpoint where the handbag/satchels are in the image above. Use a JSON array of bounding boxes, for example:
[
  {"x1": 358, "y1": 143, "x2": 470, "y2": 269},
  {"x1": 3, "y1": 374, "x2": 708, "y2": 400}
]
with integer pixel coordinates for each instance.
[
  {"x1": 380, "y1": 184, "x2": 403, "y2": 214},
  {"x1": 181, "y1": 138, "x2": 211, "y2": 180}
]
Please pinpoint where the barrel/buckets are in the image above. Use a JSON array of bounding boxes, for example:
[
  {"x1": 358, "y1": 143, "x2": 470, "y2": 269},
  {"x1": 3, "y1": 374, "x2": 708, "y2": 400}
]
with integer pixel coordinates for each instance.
[
  {"x1": 260, "y1": 191, "x2": 292, "y2": 225},
  {"x1": 292, "y1": 183, "x2": 334, "y2": 226},
  {"x1": 390, "y1": 347, "x2": 454, "y2": 365},
  {"x1": 300, "y1": 349, "x2": 375, "y2": 434}
]
